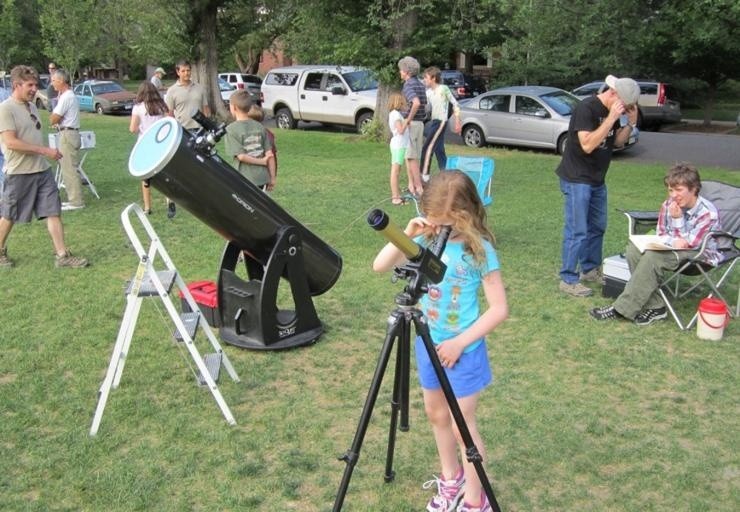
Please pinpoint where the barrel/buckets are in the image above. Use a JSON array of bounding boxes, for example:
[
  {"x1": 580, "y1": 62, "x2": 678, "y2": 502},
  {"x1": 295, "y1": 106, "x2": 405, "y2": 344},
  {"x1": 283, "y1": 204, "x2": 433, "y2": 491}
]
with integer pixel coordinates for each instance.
[{"x1": 696, "y1": 298, "x2": 729, "y2": 341}]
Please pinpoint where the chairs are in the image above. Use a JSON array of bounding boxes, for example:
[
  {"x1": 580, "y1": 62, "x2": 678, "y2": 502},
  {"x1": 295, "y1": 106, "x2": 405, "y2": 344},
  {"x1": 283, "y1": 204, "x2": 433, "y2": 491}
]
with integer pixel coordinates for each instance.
[
  {"x1": 612, "y1": 177, "x2": 740, "y2": 330},
  {"x1": 403, "y1": 154, "x2": 497, "y2": 252}
]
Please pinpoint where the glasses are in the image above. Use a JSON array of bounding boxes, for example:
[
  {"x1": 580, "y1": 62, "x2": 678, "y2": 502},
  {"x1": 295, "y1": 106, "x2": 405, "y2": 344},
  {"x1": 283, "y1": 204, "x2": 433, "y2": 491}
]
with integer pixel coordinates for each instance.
[{"x1": 30, "y1": 113, "x2": 41, "y2": 129}]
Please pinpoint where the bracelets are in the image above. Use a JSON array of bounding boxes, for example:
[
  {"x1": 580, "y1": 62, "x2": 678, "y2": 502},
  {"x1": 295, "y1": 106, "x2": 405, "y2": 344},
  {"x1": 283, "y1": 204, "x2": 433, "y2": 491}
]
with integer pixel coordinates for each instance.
[{"x1": 626, "y1": 120, "x2": 637, "y2": 130}]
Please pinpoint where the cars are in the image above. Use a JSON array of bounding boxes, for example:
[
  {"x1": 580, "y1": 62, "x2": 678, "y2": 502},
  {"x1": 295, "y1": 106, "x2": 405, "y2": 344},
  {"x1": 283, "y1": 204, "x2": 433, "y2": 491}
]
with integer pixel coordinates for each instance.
[
  {"x1": 73, "y1": 80, "x2": 138, "y2": 115},
  {"x1": 439, "y1": 70, "x2": 487, "y2": 99},
  {"x1": 217, "y1": 77, "x2": 248, "y2": 110},
  {"x1": 449, "y1": 86, "x2": 639, "y2": 157},
  {"x1": 0, "y1": 74, "x2": 14, "y2": 103},
  {"x1": 33, "y1": 77, "x2": 59, "y2": 112}
]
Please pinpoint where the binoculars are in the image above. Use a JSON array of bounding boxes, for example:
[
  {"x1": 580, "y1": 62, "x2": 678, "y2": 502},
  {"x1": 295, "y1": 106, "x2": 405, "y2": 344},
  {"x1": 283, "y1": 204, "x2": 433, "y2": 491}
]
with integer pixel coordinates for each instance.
[
  {"x1": 128, "y1": 109, "x2": 342, "y2": 351},
  {"x1": 367, "y1": 210, "x2": 452, "y2": 284}
]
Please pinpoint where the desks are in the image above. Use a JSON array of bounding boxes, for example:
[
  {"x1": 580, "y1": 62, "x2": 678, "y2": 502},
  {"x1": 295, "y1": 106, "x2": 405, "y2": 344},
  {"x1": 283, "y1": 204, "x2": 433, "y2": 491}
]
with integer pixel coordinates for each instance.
[{"x1": 48, "y1": 146, "x2": 102, "y2": 201}]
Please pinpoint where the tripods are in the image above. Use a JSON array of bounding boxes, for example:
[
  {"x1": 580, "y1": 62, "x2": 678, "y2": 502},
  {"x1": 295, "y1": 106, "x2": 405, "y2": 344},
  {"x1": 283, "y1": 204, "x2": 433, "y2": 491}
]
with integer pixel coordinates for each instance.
[{"x1": 332, "y1": 292, "x2": 502, "y2": 512}]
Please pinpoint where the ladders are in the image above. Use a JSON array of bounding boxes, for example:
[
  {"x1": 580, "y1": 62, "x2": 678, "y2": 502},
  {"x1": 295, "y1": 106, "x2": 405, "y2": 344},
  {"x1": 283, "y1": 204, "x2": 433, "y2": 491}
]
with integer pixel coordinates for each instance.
[{"x1": 88, "y1": 202, "x2": 242, "y2": 438}]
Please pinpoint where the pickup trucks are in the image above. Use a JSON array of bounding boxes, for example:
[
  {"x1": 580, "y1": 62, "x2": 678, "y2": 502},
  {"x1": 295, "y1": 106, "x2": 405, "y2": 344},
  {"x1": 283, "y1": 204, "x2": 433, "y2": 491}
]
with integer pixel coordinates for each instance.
[{"x1": 259, "y1": 65, "x2": 378, "y2": 137}]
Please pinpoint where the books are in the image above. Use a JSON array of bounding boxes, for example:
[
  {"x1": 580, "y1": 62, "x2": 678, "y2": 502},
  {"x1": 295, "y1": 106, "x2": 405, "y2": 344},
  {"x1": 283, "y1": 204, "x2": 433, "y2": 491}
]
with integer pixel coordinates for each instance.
[{"x1": 628, "y1": 234, "x2": 693, "y2": 255}]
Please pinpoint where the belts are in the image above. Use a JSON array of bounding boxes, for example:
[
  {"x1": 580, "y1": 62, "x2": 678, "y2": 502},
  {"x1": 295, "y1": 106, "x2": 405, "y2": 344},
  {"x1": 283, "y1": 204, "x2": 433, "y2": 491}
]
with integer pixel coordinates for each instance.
[{"x1": 56, "y1": 127, "x2": 78, "y2": 131}]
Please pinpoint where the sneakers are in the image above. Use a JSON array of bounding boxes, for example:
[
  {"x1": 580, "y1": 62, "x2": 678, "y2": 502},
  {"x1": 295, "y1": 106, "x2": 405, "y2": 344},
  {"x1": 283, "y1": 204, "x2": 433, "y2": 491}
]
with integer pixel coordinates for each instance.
[
  {"x1": 457, "y1": 489, "x2": 491, "y2": 511},
  {"x1": 0, "y1": 201, "x2": 175, "y2": 269},
  {"x1": 559, "y1": 267, "x2": 668, "y2": 327},
  {"x1": 422, "y1": 466, "x2": 466, "y2": 511}
]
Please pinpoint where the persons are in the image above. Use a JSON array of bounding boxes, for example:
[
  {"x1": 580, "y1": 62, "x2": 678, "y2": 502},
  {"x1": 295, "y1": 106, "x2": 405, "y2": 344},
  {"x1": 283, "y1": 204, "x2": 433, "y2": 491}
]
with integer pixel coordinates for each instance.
[
  {"x1": 587, "y1": 161, "x2": 721, "y2": 326},
  {"x1": 150, "y1": 67, "x2": 169, "y2": 100},
  {"x1": 554, "y1": 73, "x2": 641, "y2": 297},
  {"x1": 130, "y1": 81, "x2": 175, "y2": 218},
  {"x1": 46, "y1": 61, "x2": 85, "y2": 211},
  {"x1": 369, "y1": 168, "x2": 509, "y2": 512},
  {"x1": 389, "y1": 55, "x2": 461, "y2": 207},
  {"x1": 224, "y1": 90, "x2": 278, "y2": 192},
  {"x1": 1, "y1": 64, "x2": 89, "y2": 270},
  {"x1": 164, "y1": 60, "x2": 210, "y2": 134}
]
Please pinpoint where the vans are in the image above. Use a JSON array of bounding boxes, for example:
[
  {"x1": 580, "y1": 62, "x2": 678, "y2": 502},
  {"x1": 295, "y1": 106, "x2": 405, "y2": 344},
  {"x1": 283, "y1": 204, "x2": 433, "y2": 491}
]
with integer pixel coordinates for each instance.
[
  {"x1": 219, "y1": 73, "x2": 265, "y2": 101},
  {"x1": 568, "y1": 81, "x2": 682, "y2": 134}
]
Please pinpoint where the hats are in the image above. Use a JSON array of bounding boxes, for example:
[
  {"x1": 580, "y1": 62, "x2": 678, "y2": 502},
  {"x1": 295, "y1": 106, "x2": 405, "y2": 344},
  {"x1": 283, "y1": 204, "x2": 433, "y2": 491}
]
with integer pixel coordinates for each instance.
[{"x1": 605, "y1": 74, "x2": 641, "y2": 107}]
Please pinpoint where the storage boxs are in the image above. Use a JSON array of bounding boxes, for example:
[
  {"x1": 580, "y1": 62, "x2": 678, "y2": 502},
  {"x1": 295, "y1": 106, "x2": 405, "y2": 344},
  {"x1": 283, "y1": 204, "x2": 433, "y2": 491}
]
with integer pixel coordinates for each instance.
[
  {"x1": 46, "y1": 131, "x2": 97, "y2": 151},
  {"x1": 601, "y1": 252, "x2": 640, "y2": 283}
]
